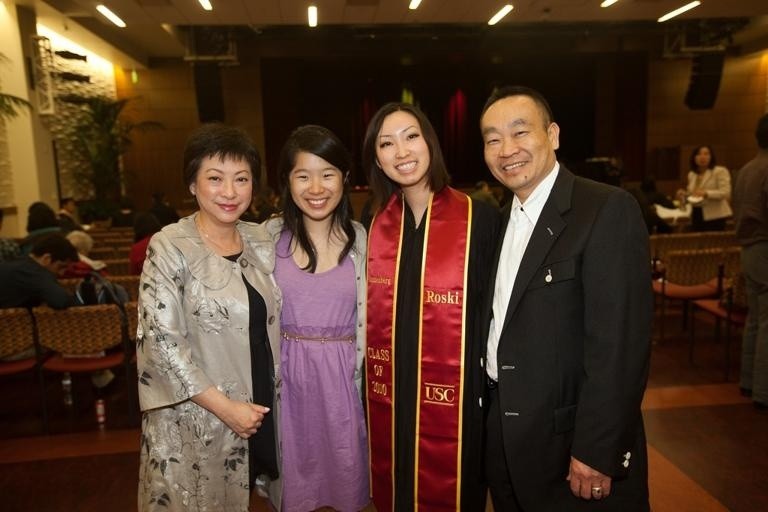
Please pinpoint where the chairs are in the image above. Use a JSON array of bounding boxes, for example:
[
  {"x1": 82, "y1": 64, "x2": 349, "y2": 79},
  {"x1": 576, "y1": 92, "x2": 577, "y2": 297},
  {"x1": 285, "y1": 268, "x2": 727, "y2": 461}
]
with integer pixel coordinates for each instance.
[
  {"x1": 608, "y1": 172, "x2": 752, "y2": 390},
  {"x1": 0, "y1": 211, "x2": 154, "y2": 440}
]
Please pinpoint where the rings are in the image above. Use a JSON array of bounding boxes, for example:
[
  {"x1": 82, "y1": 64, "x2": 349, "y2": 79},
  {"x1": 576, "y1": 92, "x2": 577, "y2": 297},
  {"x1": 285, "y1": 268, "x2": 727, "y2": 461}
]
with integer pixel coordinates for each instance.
[{"x1": 592, "y1": 487, "x2": 602, "y2": 493}]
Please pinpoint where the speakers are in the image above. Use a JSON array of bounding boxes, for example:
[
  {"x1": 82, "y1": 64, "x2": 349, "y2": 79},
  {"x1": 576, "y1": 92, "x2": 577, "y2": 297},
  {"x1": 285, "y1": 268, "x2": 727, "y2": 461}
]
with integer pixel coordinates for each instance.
[
  {"x1": 194, "y1": 66, "x2": 224, "y2": 123},
  {"x1": 684, "y1": 53, "x2": 723, "y2": 110}
]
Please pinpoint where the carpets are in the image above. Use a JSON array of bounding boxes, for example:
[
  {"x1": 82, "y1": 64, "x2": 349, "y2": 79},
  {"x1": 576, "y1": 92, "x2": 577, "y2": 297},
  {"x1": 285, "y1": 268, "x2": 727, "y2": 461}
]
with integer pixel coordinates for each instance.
[
  {"x1": 0, "y1": 446, "x2": 275, "y2": 511},
  {"x1": 637, "y1": 397, "x2": 767, "y2": 511}
]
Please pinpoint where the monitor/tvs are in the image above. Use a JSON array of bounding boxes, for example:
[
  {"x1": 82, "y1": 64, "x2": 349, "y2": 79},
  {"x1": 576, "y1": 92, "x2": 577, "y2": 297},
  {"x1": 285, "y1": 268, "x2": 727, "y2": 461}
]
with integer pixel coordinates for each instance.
[{"x1": 188, "y1": 29, "x2": 229, "y2": 54}]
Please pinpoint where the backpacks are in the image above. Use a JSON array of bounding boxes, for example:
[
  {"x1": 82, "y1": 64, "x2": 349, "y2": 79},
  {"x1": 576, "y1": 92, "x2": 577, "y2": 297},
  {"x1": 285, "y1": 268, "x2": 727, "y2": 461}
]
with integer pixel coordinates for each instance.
[{"x1": 72, "y1": 271, "x2": 130, "y2": 307}]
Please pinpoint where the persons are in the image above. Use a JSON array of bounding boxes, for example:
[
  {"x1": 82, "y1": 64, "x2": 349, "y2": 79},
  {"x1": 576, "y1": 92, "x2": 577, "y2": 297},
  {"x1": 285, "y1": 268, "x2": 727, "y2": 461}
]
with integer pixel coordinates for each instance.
[
  {"x1": 479, "y1": 88, "x2": 649, "y2": 512},
  {"x1": 587, "y1": 143, "x2": 733, "y2": 232},
  {"x1": 468, "y1": 183, "x2": 498, "y2": 205},
  {"x1": 363, "y1": 103, "x2": 499, "y2": 512},
  {"x1": 2, "y1": 190, "x2": 180, "y2": 309},
  {"x1": 244, "y1": 189, "x2": 281, "y2": 224},
  {"x1": 136, "y1": 122, "x2": 279, "y2": 512},
  {"x1": 734, "y1": 114, "x2": 767, "y2": 412},
  {"x1": 260, "y1": 125, "x2": 371, "y2": 512}
]
